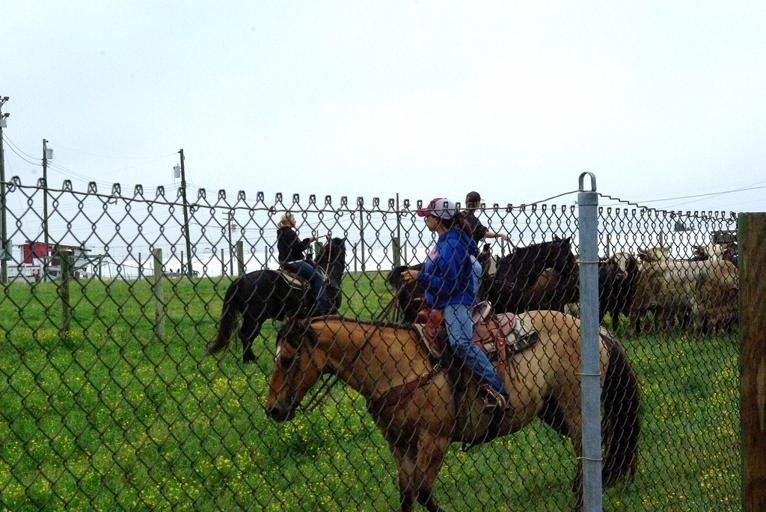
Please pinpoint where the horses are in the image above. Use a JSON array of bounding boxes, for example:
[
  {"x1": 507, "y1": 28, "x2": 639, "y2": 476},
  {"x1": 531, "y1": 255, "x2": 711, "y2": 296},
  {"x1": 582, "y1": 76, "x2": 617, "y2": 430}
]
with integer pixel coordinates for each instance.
[
  {"x1": 205, "y1": 235, "x2": 348, "y2": 364},
  {"x1": 385, "y1": 233, "x2": 580, "y2": 325},
  {"x1": 265, "y1": 309, "x2": 642, "y2": 512}
]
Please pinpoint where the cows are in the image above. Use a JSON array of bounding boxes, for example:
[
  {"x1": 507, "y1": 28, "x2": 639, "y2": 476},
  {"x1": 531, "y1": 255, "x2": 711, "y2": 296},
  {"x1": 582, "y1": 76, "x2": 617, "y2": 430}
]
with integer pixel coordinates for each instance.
[{"x1": 529, "y1": 244, "x2": 739, "y2": 342}]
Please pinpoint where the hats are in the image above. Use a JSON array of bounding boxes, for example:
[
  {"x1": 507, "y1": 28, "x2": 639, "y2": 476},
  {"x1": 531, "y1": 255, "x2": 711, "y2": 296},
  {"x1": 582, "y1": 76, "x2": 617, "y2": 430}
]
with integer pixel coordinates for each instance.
[
  {"x1": 465, "y1": 192, "x2": 484, "y2": 201},
  {"x1": 417, "y1": 199, "x2": 455, "y2": 219}
]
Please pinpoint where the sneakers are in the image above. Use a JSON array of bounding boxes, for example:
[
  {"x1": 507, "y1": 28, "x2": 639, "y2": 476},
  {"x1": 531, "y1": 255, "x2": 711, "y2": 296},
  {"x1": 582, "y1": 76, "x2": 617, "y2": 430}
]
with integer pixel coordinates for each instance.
[{"x1": 484, "y1": 391, "x2": 510, "y2": 414}]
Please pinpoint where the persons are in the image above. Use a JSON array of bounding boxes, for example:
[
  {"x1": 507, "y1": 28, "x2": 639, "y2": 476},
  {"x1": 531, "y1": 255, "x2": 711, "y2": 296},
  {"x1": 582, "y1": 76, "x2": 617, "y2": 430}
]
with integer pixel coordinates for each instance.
[
  {"x1": 32, "y1": 268, "x2": 44, "y2": 283},
  {"x1": 277, "y1": 211, "x2": 337, "y2": 315},
  {"x1": 400, "y1": 191, "x2": 511, "y2": 412}
]
